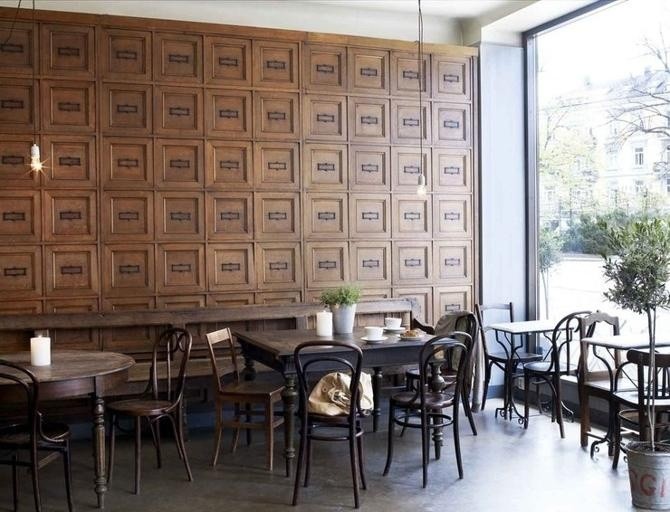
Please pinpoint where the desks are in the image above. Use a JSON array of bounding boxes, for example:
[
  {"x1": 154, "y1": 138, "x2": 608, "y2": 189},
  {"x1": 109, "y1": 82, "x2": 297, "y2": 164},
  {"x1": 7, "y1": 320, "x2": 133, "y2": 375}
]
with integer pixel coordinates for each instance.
[
  {"x1": 0, "y1": 349, "x2": 135, "y2": 510},
  {"x1": 489, "y1": 320, "x2": 574, "y2": 429},
  {"x1": 233, "y1": 326, "x2": 459, "y2": 478}
]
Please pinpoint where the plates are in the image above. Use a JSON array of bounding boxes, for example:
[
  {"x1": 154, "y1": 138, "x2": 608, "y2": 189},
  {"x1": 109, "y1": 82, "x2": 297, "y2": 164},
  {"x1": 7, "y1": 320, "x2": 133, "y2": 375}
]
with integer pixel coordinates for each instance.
[
  {"x1": 383, "y1": 327, "x2": 406, "y2": 333},
  {"x1": 361, "y1": 337, "x2": 388, "y2": 343},
  {"x1": 396, "y1": 335, "x2": 424, "y2": 341}
]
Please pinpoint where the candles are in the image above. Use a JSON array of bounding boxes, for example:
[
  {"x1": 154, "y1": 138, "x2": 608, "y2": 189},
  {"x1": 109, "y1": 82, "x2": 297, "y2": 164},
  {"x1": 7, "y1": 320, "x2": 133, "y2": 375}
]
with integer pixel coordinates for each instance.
[
  {"x1": 30, "y1": 334, "x2": 52, "y2": 365},
  {"x1": 316, "y1": 309, "x2": 333, "y2": 336}
]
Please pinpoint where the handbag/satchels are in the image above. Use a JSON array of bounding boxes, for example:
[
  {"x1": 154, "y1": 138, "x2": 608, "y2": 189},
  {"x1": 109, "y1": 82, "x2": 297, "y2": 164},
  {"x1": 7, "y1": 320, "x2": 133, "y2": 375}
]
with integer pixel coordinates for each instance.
[{"x1": 306, "y1": 372, "x2": 375, "y2": 417}]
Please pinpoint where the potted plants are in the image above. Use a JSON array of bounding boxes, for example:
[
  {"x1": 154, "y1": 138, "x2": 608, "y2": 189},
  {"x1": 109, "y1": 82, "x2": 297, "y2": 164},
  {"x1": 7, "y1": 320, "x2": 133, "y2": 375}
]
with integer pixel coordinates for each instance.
[
  {"x1": 599, "y1": 219, "x2": 670, "y2": 511},
  {"x1": 321, "y1": 285, "x2": 361, "y2": 332}
]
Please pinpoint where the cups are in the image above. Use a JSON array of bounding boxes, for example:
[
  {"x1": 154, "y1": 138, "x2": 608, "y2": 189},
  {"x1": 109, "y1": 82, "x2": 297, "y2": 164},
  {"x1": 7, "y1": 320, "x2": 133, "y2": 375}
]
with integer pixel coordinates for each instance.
[
  {"x1": 364, "y1": 326, "x2": 383, "y2": 339},
  {"x1": 384, "y1": 317, "x2": 402, "y2": 329}
]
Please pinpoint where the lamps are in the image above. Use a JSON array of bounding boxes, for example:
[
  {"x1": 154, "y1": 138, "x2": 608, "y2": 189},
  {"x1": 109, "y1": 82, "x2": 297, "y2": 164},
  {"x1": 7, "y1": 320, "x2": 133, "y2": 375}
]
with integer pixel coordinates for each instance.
[
  {"x1": 31, "y1": 0, "x2": 44, "y2": 172},
  {"x1": 415, "y1": 0, "x2": 428, "y2": 196}
]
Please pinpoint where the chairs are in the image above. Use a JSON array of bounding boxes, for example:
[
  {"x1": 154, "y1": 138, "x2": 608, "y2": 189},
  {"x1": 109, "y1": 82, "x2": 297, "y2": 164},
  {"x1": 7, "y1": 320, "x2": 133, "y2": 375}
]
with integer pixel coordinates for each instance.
[
  {"x1": 0, "y1": 359, "x2": 75, "y2": 512},
  {"x1": 475, "y1": 303, "x2": 545, "y2": 415},
  {"x1": 382, "y1": 334, "x2": 473, "y2": 487},
  {"x1": 612, "y1": 348, "x2": 670, "y2": 470},
  {"x1": 294, "y1": 340, "x2": 366, "y2": 509},
  {"x1": 524, "y1": 311, "x2": 597, "y2": 438},
  {"x1": 399, "y1": 310, "x2": 479, "y2": 436},
  {"x1": 206, "y1": 327, "x2": 284, "y2": 471},
  {"x1": 107, "y1": 328, "x2": 192, "y2": 495},
  {"x1": 579, "y1": 313, "x2": 632, "y2": 456}
]
